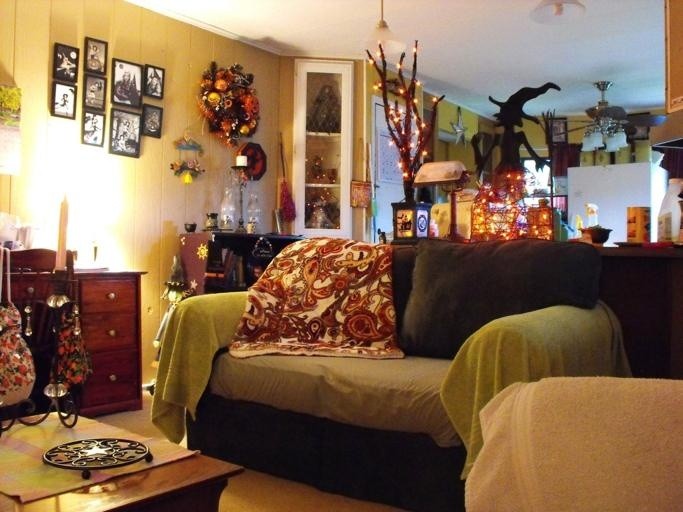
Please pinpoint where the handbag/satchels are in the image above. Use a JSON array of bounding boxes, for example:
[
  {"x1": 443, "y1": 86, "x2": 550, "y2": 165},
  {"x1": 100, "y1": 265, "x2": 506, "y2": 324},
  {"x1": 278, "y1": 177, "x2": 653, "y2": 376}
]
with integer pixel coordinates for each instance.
[
  {"x1": 52, "y1": 303, "x2": 92, "y2": 386},
  {"x1": 0, "y1": 305, "x2": 36, "y2": 406}
]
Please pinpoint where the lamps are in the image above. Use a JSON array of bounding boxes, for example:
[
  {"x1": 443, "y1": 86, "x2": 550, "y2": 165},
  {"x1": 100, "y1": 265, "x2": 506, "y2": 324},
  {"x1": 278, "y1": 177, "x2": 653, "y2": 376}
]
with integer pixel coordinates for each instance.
[
  {"x1": 579, "y1": 120, "x2": 629, "y2": 152},
  {"x1": 413, "y1": 161, "x2": 467, "y2": 241}
]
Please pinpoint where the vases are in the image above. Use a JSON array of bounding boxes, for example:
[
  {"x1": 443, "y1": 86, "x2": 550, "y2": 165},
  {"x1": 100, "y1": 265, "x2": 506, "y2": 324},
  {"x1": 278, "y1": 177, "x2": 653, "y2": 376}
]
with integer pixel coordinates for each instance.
[
  {"x1": 220, "y1": 187, "x2": 234, "y2": 230},
  {"x1": 245, "y1": 191, "x2": 261, "y2": 235}
]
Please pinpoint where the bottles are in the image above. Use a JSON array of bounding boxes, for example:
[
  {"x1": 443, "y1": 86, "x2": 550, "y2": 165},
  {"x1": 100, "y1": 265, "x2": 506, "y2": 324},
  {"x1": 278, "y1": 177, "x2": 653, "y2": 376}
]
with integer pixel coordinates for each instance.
[
  {"x1": 205, "y1": 212, "x2": 219, "y2": 231},
  {"x1": 658, "y1": 178, "x2": 683, "y2": 243},
  {"x1": 220, "y1": 187, "x2": 236, "y2": 233},
  {"x1": 377, "y1": 228, "x2": 387, "y2": 246},
  {"x1": 429, "y1": 220, "x2": 439, "y2": 238},
  {"x1": 247, "y1": 191, "x2": 260, "y2": 234}
]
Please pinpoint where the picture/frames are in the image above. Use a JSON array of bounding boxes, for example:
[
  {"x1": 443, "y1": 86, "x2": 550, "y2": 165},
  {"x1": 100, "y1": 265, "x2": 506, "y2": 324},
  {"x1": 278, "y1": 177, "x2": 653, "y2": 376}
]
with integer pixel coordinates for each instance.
[
  {"x1": 81, "y1": 111, "x2": 106, "y2": 147},
  {"x1": 142, "y1": 103, "x2": 163, "y2": 138},
  {"x1": 83, "y1": 37, "x2": 108, "y2": 76},
  {"x1": 52, "y1": 81, "x2": 77, "y2": 120},
  {"x1": 625, "y1": 111, "x2": 649, "y2": 140},
  {"x1": 545, "y1": 117, "x2": 568, "y2": 144},
  {"x1": 144, "y1": 64, "x2": 165, "y2": 98},
  {"x1": 108, "y1": 108, "x2": 142, "y2": 158},
  {"x1": 83, "y1": 73, "x2": 107, "y2": 112},
  {"x1": 111, "y1": 58, "x2": 144, "y2": 109},
  {"x1": 52, "y1": 43, "x2": 79, "y2": 83}
]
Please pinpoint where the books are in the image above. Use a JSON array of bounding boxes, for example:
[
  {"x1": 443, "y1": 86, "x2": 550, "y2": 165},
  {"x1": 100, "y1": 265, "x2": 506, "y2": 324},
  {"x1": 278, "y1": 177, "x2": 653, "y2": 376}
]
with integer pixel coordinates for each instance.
[{"x1": 205, "y1": 247, "x2": 243, "y2": 289}]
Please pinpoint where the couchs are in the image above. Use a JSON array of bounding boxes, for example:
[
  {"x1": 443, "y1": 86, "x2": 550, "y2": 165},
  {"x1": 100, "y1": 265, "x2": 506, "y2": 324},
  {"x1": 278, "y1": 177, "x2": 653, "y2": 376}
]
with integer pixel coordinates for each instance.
[
  {"x1": 463, "y1": 377, "x2": 682, "y2": 512},
  {"x1": 151, "y1": 238, "x2": 635, "y2": 511}
]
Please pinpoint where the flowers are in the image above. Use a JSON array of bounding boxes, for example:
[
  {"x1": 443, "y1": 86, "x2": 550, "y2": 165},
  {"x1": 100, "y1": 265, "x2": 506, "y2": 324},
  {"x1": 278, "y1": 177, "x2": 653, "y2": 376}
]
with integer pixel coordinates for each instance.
[
  {"x1": 196, "y1": 60, "x2": 260, "y2": 148},
  {"x1": 169, "y1": 159, "x2": 203, "y2": 183}
]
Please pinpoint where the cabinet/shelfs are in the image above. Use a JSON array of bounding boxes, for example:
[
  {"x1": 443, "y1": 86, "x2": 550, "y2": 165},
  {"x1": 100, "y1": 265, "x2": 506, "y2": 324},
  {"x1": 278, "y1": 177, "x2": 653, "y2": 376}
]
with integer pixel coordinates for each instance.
[
  {"x1": 179, "y1": 231, "x2": 301, "y2": 299},
  {"x1": 1, "y1": 271, "x2": 75, "y2": 305},
  {"x1": 292, "y1": 57, "x2": 354, "y2": 240},
  {"x1": 75, "y1": 271, "x2": 147, "y2": 418}
]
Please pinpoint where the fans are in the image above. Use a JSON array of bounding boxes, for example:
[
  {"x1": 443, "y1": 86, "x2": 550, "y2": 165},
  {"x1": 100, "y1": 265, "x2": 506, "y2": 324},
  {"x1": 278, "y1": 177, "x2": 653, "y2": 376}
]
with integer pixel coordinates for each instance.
[{"x1": 537, "y1": 81, "x2": 667, "y2": 153}]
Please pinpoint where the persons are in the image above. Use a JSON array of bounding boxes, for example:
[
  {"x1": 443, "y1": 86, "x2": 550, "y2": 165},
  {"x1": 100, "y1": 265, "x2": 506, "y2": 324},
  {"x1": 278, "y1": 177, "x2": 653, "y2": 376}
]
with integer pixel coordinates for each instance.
[{"x1": 55, "y1": 43, "x2": 160, "y2": 151}]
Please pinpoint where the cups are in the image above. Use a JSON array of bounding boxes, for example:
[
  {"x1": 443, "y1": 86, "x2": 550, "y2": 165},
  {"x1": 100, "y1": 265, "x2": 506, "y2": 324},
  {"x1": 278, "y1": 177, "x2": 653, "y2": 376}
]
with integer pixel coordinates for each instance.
[
  {"x1": 17, "y1": 224, "x2": 36, "y2": 249},
  {"x1": 185, "y1": 224, "x2": 197, "y2": 232},
  {"x1": 527, "y1": 208, "x2": 563, "y2": 241},
  {"x1": 627, "y1": 206, "x2": 651, "y2": 243}
]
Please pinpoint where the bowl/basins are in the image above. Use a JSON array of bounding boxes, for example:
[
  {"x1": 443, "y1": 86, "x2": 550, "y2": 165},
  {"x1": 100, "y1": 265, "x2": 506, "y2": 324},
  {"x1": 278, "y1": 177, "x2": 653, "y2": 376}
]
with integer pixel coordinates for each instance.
[{"x1": 579, "y1": 228, "x2": 613, "y2": 243}]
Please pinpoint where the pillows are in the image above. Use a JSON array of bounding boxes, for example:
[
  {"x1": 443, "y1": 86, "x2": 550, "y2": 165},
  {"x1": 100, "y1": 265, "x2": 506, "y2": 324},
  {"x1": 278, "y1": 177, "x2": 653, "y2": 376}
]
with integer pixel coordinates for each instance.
[{"x1": 395, "y1": 237, "x2": 603, "y2": 361}]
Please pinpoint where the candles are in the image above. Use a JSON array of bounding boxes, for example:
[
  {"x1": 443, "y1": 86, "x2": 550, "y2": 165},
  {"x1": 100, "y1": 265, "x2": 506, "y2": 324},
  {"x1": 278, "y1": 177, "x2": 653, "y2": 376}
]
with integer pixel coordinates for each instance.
[
  {"x1": 235, "y1": 155, "x2": 248, "y2": 166},
  {"x1": 54, "y1": 195, "x2": 68, "y2": 270}
]
